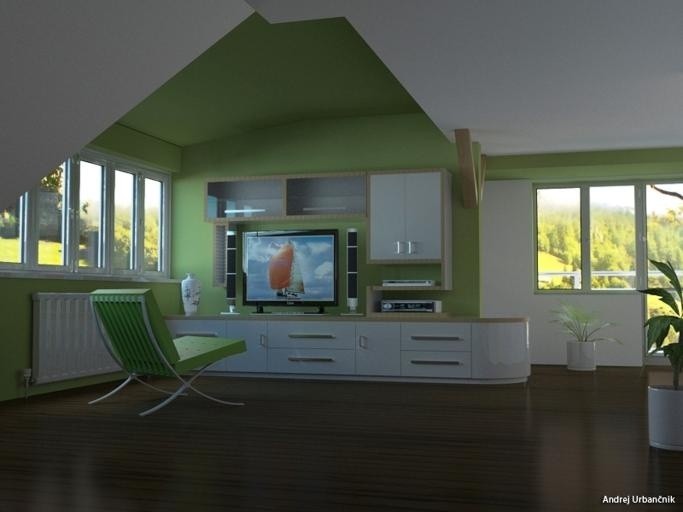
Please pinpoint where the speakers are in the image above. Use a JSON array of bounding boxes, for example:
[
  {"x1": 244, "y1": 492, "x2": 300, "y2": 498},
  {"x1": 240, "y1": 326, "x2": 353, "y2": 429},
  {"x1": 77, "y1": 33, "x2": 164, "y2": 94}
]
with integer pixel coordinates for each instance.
[
  {"x1": 341, "y1": 228, "x2": 363, "y2": 315},
  {"x1": 219, "y1": 231, "x2": 240, "y2": 314}
]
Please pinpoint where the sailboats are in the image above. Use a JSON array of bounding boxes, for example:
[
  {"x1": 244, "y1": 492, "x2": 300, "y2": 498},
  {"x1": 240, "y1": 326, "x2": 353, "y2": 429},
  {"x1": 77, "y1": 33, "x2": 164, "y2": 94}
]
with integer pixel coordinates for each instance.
[{"x1": 267, "y1": 240, "x2": 305, "y2": 299}]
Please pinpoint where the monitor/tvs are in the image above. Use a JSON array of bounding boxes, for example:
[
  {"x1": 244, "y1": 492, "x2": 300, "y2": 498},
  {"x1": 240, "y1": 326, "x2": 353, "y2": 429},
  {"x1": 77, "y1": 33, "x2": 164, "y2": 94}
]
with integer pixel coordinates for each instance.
[{"x1": 242, "y1": 229, "x2": 339, "y2": 316}]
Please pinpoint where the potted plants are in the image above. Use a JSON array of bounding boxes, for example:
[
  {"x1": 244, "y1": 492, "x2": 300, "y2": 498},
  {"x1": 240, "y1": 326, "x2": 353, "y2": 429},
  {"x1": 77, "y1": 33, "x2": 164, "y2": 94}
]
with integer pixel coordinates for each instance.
[
  {"x1": 547, "y1": 300, "x2": 623, "y2": 373},
  {"x1": 634, "y1": 256, "x2": 683, "y2": 452}
]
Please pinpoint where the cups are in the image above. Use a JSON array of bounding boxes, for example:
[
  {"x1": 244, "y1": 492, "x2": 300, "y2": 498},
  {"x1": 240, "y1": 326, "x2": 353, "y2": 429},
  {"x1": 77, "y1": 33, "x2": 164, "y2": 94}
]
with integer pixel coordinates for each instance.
[
  {"x1": 216, "y1": 200, "x2": 225, "y2": 217},
  {"x1": 243, "y1": 206, "x2": 256, "y2": 217},
  {"x1": 226, "y1": 200, "x2": 236, "y2": 218}
]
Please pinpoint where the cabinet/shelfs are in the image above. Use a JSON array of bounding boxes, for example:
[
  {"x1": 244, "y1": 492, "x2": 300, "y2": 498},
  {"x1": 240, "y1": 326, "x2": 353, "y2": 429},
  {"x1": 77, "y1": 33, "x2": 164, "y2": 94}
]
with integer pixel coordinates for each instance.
[
  {"x1": 364, "y1": 264, "x2": 452, "y2": 314},
  {"x1": 366, "y1": 165, "x2": 453, "y2": 268},
  {"x1": 198, "y1": 171, "x2": 366, "y2": 223},
  {"x1": 161, "y1": 310, "x2": 531, "y2": 387}
]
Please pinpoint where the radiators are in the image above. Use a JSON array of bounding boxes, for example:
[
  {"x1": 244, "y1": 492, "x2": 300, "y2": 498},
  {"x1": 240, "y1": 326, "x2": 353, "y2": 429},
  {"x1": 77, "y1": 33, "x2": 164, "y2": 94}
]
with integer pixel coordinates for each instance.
[{"x1": 23, "y1": 290, "x2": 125, "y2": 386}]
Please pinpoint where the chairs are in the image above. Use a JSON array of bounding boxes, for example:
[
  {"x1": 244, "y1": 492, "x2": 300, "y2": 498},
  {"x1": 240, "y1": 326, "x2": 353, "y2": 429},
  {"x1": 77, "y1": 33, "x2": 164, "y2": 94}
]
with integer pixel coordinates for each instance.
[{"x1": 86, "y1": 285, "x2": 249, "y2": 417}]
